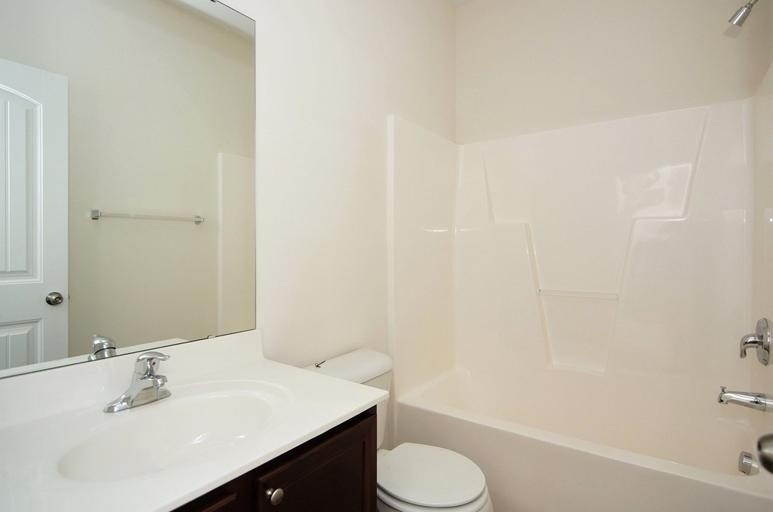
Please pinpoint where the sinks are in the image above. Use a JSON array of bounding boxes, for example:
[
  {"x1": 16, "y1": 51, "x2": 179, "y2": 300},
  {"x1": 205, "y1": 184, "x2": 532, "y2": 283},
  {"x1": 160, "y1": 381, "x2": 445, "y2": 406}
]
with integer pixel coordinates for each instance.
[{"x1": 53, "y1": 382, "x2": 321, "y2": 499}]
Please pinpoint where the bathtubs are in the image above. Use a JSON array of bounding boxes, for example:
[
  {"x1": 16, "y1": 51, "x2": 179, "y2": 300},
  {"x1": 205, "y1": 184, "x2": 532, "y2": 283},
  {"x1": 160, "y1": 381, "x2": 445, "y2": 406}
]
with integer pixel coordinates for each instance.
[{"x1": 396, "y1": 366, "x2": 773, "y2": 512}]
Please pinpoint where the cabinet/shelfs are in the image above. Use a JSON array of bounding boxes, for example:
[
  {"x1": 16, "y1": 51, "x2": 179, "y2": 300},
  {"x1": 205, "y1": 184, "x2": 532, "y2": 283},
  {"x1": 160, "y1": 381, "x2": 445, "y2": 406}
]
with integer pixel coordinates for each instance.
[{"x1": 180, "y1": 407, "x2": 378, "y2": 510}]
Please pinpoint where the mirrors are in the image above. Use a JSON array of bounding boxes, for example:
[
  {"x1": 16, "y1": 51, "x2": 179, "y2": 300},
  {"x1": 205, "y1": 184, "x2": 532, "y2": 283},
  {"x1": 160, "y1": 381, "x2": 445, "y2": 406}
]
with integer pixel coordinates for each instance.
[{"x1": 0, "y1": 0, "x2": 263, "y2": 379}]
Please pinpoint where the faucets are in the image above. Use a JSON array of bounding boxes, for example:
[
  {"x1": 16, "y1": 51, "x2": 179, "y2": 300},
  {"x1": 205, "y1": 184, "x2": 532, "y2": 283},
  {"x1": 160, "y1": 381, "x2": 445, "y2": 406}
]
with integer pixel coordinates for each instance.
[
  {"x1": 85, "y1": 335, "x2": 117, "y2": 361},
  {"x1": 715, "y1": 388, "x2": 773, "y2": 415},
  {"x1": 104, "y1": 352, "x2": 173, "y2": 413}
]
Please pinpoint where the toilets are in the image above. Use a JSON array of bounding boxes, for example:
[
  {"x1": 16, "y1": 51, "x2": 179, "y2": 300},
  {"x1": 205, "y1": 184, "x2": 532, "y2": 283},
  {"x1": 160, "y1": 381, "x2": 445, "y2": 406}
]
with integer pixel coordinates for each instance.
[{"x1": 304, "y1": 346, "x2": 494, "y2": 512}]
